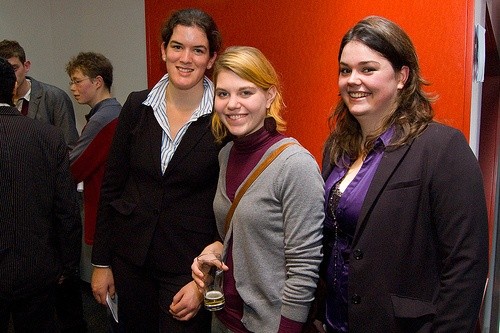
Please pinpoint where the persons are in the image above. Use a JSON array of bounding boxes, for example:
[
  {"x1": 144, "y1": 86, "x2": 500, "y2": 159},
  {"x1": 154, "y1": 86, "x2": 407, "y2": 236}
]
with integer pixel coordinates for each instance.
[
  {"x1": 310, "y1": 16, "x2": 489, "y2": 333},
  {"x1": 192, "y1": 46, "x2": 326, "y2": 333},
  {"x1": 90, "y1": 8, "x2": 232, "y2": 333},
  {"x1": 0, "y1": 40, "x2": 81, "y2": 333},
  {"x1": 68, "y1": 51, "x2": 122, "y2": 333}
]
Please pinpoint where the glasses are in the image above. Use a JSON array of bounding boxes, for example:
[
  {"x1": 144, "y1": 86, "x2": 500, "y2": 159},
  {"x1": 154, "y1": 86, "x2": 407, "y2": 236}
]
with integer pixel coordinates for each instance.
[{"x1": 68, "y1": 76, "x2": 96, "y2": 87}]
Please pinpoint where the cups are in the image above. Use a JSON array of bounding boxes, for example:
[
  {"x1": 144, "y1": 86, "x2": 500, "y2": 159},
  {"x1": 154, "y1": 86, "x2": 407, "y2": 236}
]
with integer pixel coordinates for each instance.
[{"x1": 197, "y1": 253, "x2": 226, "y2": 312}]
